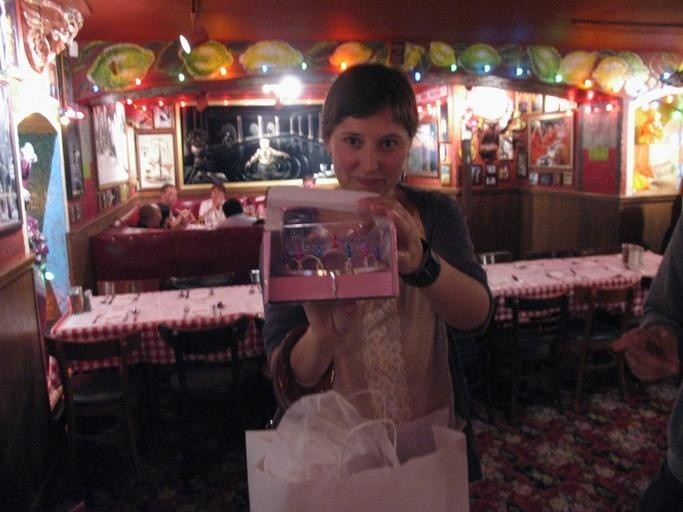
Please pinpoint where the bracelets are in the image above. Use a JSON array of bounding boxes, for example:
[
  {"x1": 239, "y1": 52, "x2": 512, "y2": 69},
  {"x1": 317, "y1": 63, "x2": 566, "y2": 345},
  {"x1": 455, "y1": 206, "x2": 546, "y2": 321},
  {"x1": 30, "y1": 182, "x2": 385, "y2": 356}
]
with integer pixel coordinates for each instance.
[{"x1": 399, "y1": 237, "x2": 440, "y2": 288}]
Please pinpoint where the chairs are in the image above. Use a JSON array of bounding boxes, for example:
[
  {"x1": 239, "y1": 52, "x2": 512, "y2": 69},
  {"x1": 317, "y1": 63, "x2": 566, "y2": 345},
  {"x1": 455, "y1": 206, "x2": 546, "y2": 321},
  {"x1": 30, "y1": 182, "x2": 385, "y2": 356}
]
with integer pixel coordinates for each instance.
[
  {"x1": 157, "y1": 316, "x2": 249, "y2": 454},
  {"x1": 482, "y1": 290, "x2": 567, "y2": 425},
  {"x1": 475, "y1": 247, "x2": 596, "y2": 265},
  {"x1": 46, "y1": 328, "x2": 155, "y2": 477},
  {"x1": 567, "y1": 284, "x2": 638, "y2": 413},
  {"x1": 159, "y1": 272, "x2": 244, "y2": 291}
]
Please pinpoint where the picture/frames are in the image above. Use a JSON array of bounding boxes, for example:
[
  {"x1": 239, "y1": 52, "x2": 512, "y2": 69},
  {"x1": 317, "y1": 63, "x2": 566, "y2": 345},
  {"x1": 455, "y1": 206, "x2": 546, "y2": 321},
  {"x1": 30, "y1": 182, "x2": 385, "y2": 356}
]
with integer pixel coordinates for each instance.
[{"x1": 526, "y1": 110, "x2": 576, "y2": 175}]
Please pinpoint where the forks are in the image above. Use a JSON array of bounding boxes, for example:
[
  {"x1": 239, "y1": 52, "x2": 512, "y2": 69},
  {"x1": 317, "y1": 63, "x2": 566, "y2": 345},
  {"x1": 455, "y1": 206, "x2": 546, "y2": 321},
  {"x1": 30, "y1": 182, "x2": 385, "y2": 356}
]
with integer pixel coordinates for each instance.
[{"x1": 131, "y1": 307, "x2": 140, "y2": 322}]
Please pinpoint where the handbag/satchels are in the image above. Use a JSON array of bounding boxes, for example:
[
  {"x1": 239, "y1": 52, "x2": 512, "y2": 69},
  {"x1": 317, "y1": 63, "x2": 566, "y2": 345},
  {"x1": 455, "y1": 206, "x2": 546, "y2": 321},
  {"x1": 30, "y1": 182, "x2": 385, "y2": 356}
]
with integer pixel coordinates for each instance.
[{"x1": 246, "y1": 387, "x2": 470, "y2": 511}]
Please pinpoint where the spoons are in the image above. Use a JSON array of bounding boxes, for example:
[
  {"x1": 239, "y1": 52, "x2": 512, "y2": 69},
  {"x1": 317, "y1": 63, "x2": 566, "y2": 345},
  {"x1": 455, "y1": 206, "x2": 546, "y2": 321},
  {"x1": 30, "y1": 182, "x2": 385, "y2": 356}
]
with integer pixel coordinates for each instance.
[{"x1": 216, "y1": 301, "x2": 227, "y2": 318}]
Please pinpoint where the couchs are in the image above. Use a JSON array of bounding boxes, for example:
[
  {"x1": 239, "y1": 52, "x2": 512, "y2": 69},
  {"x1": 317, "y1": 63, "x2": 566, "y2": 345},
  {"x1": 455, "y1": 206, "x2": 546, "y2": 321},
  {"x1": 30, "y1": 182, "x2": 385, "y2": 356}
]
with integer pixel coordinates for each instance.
[{"x1": 93, "y1": 194, "x2": 266, "y2": 295}]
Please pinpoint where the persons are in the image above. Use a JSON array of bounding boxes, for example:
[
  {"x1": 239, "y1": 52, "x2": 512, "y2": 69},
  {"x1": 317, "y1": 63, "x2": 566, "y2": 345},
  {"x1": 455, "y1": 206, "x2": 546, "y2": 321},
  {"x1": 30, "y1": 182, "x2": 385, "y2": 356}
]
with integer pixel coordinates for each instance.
[
  {"x1": 245, "y1": 138, "x2": 290, "y2": 169},
  {"x1": 135, "y1": 183, "x2": 265, "y2": 229},
  {"x1": 265, "y1": 62, "x2": 496, "y2": 431},
  {"x1": 613, "y1": 215, "x2": 683, "y2": 511}
]
